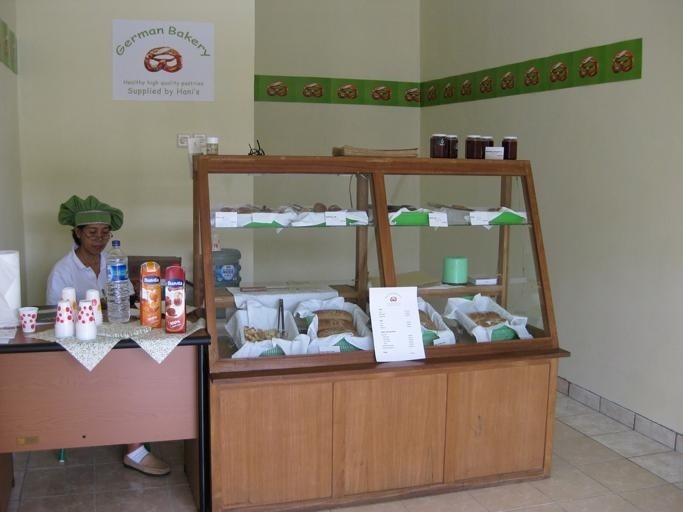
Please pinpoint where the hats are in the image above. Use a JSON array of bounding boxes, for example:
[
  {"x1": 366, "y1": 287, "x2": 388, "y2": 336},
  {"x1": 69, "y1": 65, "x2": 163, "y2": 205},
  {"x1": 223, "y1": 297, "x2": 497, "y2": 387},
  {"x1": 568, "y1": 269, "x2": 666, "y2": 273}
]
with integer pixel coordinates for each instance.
[{"x1": 58, "y1": 195, "x2": 123, "y2": 231}]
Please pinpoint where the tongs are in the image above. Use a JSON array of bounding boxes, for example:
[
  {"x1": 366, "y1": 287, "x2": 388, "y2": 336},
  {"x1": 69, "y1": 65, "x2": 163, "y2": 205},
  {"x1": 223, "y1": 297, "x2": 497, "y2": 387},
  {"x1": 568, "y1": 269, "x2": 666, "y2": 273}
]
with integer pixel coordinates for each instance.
[{"x1": 277, "y1": 299, "x2": 285, "y2": 339}]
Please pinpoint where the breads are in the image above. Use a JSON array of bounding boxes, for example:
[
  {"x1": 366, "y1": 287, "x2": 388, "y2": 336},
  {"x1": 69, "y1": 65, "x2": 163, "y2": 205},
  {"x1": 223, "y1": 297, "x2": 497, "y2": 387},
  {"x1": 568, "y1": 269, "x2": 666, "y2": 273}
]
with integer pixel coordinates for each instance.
[
  {"x1": 313, "y1": 309, "x2": 359, "y2": 338},
  {"x1": 419, "y1": 310, "x2": 436, "y2": 330},
  {"x1": 469, "y1": 311, "x2": 507, "y2": 327}
]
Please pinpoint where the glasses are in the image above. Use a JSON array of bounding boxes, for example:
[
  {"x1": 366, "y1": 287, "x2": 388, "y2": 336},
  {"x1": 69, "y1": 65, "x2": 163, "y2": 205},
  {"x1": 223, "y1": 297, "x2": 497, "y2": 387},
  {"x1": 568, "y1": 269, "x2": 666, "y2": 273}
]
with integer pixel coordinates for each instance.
[{"x1": 83, "y1": 231, "x2": 113, "y2": 241}]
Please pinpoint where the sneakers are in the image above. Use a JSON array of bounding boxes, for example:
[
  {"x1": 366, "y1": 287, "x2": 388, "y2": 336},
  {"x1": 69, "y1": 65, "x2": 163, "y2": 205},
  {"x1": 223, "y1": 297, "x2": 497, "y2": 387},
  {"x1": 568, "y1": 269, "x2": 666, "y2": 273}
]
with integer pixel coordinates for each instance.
[{"x1": 123, "y1": 452, "x2": 171, "y2": 477}]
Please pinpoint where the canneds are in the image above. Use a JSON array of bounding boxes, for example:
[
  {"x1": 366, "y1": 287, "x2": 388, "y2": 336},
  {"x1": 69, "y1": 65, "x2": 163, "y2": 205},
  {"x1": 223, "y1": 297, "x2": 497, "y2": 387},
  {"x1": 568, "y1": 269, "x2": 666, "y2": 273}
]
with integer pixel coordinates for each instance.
[{"x1": 430, "y1": 133, "x2": 518, "y2": 160}]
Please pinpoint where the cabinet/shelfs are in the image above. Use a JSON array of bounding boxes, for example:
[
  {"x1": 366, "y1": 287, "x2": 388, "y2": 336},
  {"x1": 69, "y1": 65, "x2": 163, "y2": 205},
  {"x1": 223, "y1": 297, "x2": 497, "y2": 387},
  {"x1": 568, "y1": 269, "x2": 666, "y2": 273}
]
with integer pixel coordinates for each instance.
[{"x1": 192, "y1": 153, "x2": 570, "y2": 512}]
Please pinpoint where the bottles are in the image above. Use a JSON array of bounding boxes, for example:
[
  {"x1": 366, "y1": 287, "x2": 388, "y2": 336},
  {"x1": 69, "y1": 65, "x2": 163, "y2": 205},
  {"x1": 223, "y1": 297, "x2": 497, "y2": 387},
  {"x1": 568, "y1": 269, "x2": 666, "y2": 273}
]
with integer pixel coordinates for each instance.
[
  {"x1": 206, "y1": 136, "x2": 218, "y2": 155},
  {"x1": 430, "y1": 134, "x2": 517, "y2": 160},
  {"x1": 106, "y1": 241, "x2": 131, "y2": 323}
]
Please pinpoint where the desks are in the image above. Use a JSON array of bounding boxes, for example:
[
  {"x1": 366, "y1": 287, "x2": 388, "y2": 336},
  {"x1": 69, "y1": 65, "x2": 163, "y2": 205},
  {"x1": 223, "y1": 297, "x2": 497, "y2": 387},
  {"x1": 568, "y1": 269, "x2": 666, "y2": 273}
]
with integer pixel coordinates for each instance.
[{"x1": 0, "y1": 309, "x2": 210, "y2": 512}]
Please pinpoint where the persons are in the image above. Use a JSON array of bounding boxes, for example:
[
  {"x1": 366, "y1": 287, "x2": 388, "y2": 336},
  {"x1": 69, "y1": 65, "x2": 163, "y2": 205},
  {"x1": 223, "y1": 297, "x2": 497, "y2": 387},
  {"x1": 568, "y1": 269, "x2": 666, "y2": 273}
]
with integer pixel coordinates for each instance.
[{"x1": 45, "y1": 195, "x2": 172, "y2": 477}]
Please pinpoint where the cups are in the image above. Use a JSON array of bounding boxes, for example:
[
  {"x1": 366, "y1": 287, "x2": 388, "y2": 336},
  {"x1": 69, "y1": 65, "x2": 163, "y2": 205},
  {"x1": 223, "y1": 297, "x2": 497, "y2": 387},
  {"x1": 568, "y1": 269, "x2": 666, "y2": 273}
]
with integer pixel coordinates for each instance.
[
  {"x1": 212, "y1": 240, "x2": 221, "y2": 252},
  {"x1": 54, "y1": 287, "x2": 104, "y2": 340},
  {"x1": 17, "y1": 307, "x2": 38, "y2": 333}
]
